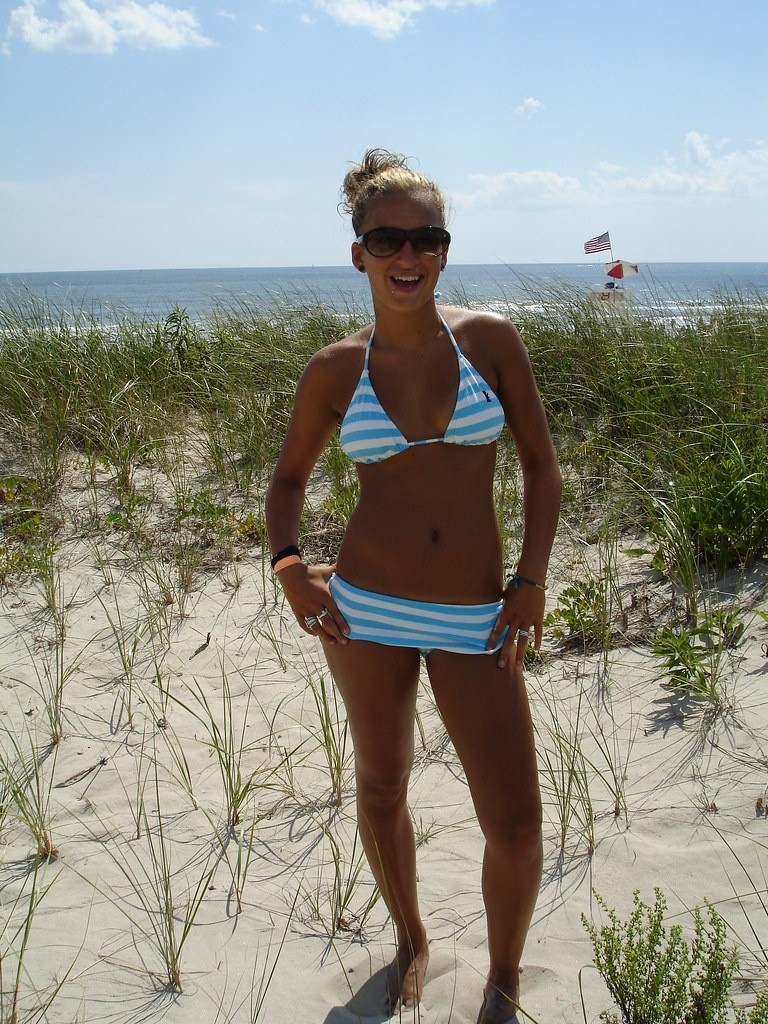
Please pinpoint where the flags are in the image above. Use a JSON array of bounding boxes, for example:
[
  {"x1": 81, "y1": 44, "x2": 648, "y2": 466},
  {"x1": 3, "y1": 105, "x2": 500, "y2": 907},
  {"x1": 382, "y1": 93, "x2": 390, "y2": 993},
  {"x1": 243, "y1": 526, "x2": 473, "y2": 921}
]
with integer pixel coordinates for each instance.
[{"x1": 584, "y1": 232, "x2": 611, "y2": 254}]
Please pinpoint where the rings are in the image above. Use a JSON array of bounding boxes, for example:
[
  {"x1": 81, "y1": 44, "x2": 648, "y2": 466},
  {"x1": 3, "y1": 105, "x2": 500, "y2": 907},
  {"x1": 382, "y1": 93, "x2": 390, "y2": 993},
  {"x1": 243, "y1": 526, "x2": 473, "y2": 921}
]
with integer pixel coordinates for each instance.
[
  {"x1": 319, "y1": 609, "x2": 329, "y2": 619},
  {"x1": 519, "y1": 630, "x2": 530, "y2": 637},
  {"x1": 305, "y1": 617, "x2": 317, "y2": 628}
]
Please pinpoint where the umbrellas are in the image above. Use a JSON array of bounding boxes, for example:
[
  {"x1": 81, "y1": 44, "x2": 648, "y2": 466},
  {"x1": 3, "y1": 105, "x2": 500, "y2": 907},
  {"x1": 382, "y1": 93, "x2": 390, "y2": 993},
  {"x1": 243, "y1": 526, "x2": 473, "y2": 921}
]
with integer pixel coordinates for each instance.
[{"x1": 606, "y1": 260, "x2": 639, "y2": 279}]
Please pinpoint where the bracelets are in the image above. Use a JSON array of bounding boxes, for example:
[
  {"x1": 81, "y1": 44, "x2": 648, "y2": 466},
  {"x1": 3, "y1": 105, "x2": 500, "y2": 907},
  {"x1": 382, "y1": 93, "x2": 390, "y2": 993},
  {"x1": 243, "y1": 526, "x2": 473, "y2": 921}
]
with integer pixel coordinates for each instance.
[
  {"x1": 509, "y1": 572, "x2": 549, "y2": 591},
  {"x1": 271, "y1": 546, "x2": 302, "y2": 575}
]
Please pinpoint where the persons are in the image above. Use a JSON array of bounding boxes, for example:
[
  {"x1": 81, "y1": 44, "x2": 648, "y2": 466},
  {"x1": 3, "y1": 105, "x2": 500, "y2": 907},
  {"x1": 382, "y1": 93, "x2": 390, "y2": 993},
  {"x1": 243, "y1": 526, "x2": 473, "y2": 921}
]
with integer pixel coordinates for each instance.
[{"x1": 267, "y1": 149, "x2": 562, "y2": 1024}]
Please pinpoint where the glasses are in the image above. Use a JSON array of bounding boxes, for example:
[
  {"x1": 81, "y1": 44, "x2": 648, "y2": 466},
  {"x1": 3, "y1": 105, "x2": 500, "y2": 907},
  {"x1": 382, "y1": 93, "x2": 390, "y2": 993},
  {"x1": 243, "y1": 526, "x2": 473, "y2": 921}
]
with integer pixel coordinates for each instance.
[{"x1": 355, "y1": 226, "x2": 451, "y2": 257}]
